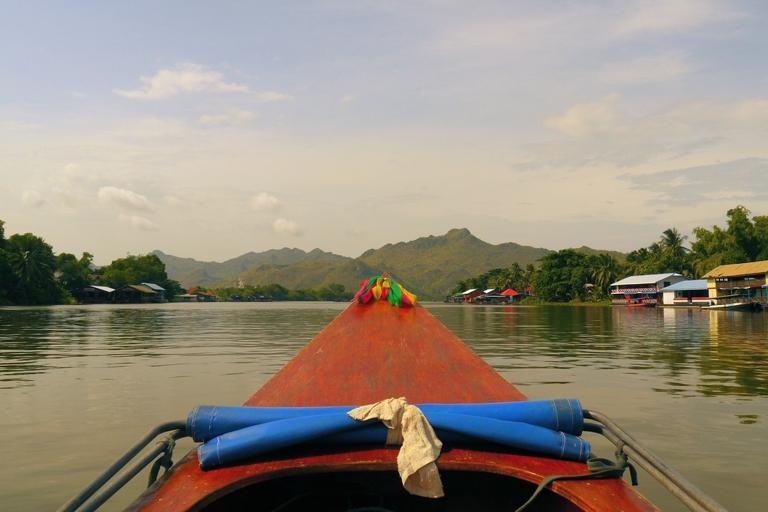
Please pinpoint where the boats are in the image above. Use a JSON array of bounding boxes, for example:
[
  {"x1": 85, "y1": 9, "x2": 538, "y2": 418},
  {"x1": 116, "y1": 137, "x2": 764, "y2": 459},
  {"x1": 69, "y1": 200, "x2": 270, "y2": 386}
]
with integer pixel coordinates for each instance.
[
  {"x1": 624, "y1": 296, "x2": 658, "y2": 309},
  {"x1": 701, "y1": 292, "x2": 762, "y2": 311}
]
